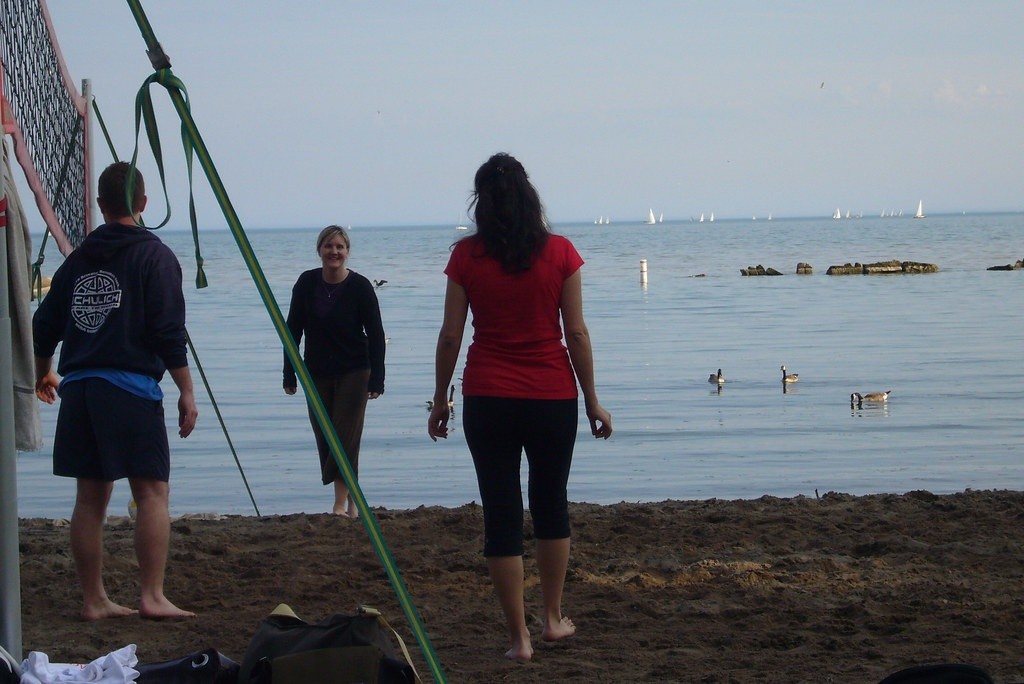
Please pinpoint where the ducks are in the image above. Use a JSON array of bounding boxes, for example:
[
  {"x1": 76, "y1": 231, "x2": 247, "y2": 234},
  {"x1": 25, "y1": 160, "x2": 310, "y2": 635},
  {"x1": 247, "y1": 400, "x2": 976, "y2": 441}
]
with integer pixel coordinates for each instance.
[
  {"x1": 707, "y1": 368, "x2": 724, "y2": 383},
  {"x1": 779, "y1": 365, "x2": 800, "y2": 383},
  {"x1": 850, "y1": 390, "x2": 892, "y2": 403}
]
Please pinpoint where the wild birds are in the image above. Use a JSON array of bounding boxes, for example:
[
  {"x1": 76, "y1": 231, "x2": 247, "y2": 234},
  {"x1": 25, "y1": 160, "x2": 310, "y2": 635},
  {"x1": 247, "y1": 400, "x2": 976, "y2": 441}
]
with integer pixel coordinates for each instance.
[{"x1": 373, "y1": 279, "x2": 388, "y2": 286}]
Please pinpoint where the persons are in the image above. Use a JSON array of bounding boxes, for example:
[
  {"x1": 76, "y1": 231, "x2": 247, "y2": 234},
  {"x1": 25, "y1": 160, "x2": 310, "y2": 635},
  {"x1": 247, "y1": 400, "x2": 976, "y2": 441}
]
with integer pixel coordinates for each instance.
[
  {"x1": 33, "y1": 162, "x2": 199, "y2": 617},
  {"x1": 427, "y1": 153, "x2": 612, "y2": 657},
  {"x1": 283, "y1": 226, "x2": 385, "y2": 517}
]
